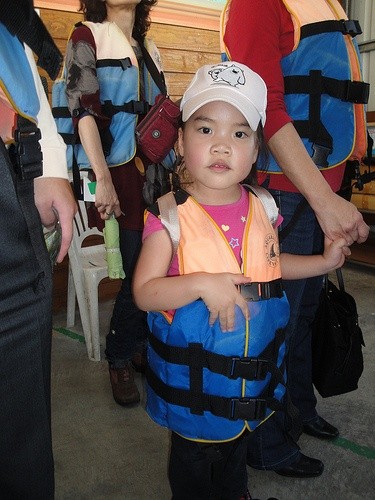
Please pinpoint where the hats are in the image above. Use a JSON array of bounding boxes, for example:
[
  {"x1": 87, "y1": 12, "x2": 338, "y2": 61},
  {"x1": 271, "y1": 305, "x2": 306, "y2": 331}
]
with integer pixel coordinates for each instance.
[{"x1": 180, "y1": 61, "x2": 268, "y2": 132}]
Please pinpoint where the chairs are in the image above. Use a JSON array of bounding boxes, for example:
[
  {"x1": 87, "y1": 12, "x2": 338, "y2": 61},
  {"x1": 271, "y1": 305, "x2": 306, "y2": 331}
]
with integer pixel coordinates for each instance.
[{"x1": 66, "y1": 170, "x2": 108, "y2": 361}]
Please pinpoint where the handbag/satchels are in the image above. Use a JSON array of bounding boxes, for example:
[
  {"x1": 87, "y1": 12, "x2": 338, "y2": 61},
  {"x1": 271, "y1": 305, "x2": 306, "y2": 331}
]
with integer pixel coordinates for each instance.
[
  {"x1": 134, "y1": 92, "x2": 180, "y2": 163},
  {"x1": 312, "y1": 248, "x2": 366, "y2": 399}
]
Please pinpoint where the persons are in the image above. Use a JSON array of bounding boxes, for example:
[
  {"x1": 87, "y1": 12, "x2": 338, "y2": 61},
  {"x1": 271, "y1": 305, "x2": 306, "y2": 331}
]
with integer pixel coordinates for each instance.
[
  {"x1": 132, "y1": 61, "x2": 352, "y2": 500},
  {"x1": 0, "y1": 0, "x2": 78, "y2": 500},
  {"x1": 65, "y1": 0, "x2": 185, "y2": 408},
  {"x1": 220, "y1": 0, "x2": 371, "y2": 480}
]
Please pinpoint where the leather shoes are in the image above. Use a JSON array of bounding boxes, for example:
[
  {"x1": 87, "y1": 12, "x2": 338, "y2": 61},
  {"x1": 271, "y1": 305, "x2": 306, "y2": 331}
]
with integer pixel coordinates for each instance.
[
  {"x1": 302, "y1": 414, "x2": 339, "y2": 441},
  {"x1": 273, "y1": 451, "x2": 324, "y2": 478}
]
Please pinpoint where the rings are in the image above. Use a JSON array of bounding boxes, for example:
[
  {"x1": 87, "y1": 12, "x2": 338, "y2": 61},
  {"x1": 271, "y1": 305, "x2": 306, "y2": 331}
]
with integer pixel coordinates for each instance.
[{"x1": 105, "y1": 212, "x2": 113, "y2": 215}]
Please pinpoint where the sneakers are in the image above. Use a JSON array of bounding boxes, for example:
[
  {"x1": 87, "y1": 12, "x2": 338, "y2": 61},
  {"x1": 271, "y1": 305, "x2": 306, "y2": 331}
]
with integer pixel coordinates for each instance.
[{"x1": 108, "y1": 356, "x2": 141, "y2": 408}]
